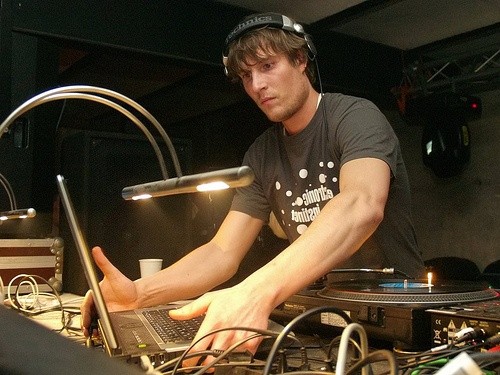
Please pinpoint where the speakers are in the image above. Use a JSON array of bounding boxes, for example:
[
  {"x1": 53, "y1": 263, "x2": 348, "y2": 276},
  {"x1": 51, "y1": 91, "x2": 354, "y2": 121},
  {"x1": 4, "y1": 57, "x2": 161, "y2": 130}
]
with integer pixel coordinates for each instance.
[{"x1": 422, "y1": 119, "x2": 473, "y2": 178}]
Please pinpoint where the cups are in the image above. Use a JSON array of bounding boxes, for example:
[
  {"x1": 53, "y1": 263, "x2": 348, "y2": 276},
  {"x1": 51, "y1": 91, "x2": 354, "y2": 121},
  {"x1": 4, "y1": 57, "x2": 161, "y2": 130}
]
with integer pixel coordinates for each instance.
[{"x1": 139, "y1": 259, "x2": 163, "y2": 279}]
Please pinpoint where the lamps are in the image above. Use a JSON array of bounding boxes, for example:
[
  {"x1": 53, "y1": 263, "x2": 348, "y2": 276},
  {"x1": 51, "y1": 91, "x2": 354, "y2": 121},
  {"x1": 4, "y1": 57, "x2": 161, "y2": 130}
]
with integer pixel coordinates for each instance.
[
  {"x1": 0, "y1": 173, "x2": 36, "y2": 221},
  {"x1": 0, "y1": 85, "x2": 254, "y2": 203}
]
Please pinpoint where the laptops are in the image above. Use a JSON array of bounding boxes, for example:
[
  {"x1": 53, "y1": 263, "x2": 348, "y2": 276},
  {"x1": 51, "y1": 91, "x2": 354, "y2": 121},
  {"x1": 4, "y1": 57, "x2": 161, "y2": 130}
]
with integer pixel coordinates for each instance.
[{"x1": 57, "y1": 174, "x2": 295, "y2": 366}]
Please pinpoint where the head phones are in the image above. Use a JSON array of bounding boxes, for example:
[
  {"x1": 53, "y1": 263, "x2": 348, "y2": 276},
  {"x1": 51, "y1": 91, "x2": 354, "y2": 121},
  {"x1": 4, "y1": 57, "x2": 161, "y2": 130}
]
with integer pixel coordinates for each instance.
[{"x1": 222, "y1": 11, "x2": 317, "y2": 96}]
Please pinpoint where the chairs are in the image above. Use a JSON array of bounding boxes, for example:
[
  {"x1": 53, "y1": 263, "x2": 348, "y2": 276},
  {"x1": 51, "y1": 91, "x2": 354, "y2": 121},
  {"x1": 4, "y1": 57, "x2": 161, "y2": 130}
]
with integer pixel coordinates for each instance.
[{"x1": 423, "y1": 257, "x2": 481, "y2": 282}]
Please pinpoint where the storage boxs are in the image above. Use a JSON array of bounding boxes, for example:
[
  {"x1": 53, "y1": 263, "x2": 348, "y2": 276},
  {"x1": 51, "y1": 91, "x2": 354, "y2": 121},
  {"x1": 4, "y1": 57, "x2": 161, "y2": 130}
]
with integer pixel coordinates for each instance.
[{"x1": 0, "y1": 237, "x2": 64, "y2": 294}]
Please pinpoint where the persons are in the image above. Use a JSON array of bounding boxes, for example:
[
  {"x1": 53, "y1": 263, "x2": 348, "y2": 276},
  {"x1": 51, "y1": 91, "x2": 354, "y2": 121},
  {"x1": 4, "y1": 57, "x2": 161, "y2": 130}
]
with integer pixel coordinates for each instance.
[{"x1": 80, "y1": 12, "x2": 427, "y2": 373}]
